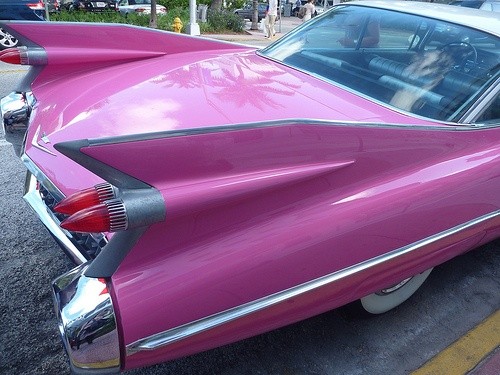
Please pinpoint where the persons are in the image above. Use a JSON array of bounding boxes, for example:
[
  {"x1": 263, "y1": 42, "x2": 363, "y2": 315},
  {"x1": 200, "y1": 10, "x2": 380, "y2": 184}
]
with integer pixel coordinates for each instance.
[
  {"x1": 336, "y1": 13, "x2": 380, "y2": 48},
  {"x1": 263, "y1": 0, "x2": 277, "y2": 38},
  {"x1": 389, "y1": 44, "x2": 464, "y2": 112},
  {"x1": 303, "y1": 0, "x2": 315, "y2": 22}
]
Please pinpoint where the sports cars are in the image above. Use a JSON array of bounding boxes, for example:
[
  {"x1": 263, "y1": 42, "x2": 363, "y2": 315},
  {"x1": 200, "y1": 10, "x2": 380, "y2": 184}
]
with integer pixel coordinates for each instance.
[{"x1": 0, "y1": 0, "x2": 500, "y2": 375}]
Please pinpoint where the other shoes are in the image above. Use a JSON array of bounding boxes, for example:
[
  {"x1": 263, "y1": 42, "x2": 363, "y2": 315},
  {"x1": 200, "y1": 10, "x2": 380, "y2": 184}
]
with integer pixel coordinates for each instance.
[
  {"x1": 265, "y1": 36, "x2": 270, "y2": 39},
  {"x1": 273, "y1": 32, "x2": 277, "y2": 36}
]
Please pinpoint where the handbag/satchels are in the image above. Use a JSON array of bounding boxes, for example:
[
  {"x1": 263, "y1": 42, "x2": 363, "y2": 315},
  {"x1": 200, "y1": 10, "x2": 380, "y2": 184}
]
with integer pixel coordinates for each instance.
[
  {"x1": 298, "y1": 7, "x2": 307, "y2": 19},
  {"x1": 259, "y1": 18, "x2": 266, "y2": 30}
]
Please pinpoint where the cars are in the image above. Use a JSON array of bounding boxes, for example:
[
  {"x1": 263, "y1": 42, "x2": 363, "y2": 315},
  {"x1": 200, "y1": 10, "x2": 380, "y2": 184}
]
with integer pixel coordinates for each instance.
[
  {"x1": 47, "y1": 0, "x2": 61, "y2": 16},
  {"x1": 116, "y1": 0, "x2": 167, "y2": 15},
  {"x1": 292, "y1": 0, "x2": 326, "y2": 19},
  {"x1": 1, "y1": 0, "x2": 47, "y2": 52},
  {"x1": 417, "y1": 0, "x2": 500, "y2": 49},
  {"x1": 60, "y1": 0, "x2": 120, "y2": 14},
  {"x1": 233, "y1": 2, "x2": 277, "y2": 22}
]
